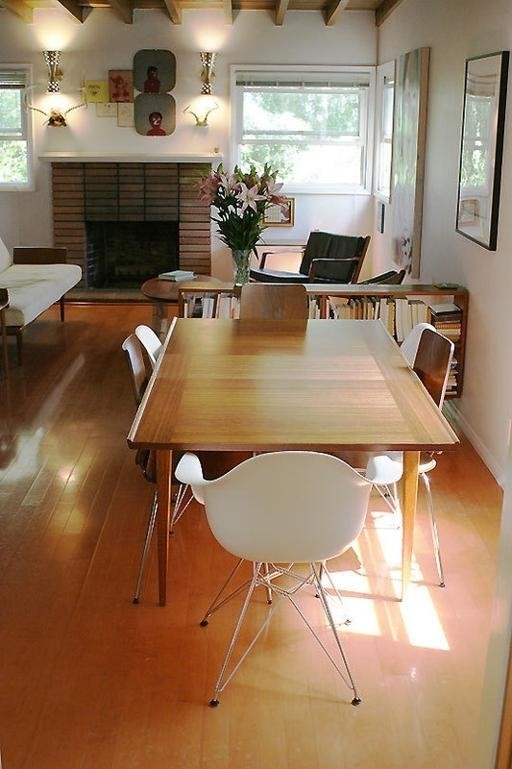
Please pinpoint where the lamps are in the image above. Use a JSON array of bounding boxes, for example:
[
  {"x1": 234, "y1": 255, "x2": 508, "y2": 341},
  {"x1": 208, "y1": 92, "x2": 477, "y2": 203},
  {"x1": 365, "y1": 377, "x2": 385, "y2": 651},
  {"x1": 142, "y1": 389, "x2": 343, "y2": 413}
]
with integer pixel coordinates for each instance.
[
  {"x1": 199, "y1": 51, "x2": 218, "y2": 94},
  {"x1": 42, "y1": 50, "x2": 64, "y2": 96}
]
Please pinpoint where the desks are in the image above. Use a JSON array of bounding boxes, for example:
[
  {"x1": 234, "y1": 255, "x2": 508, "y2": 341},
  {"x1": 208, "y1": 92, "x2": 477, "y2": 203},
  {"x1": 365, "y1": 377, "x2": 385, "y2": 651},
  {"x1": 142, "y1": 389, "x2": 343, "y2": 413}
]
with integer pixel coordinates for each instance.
[{"x1": 126, "y1": 317, "x2": 460, "y2": 606}]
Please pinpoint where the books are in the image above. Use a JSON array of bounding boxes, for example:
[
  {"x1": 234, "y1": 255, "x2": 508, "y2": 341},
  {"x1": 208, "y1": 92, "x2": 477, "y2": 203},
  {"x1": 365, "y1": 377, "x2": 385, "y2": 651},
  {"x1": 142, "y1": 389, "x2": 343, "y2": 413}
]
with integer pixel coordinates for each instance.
[
  {"x1": 308, "y1": 296, "x2": 462, "y2": 395},
  {"x1": 183, "y1": 292, "x2": 240, "y2": 320},
  {"x1": 158, "y1": 270, "x2": 194, "y2": 281}
]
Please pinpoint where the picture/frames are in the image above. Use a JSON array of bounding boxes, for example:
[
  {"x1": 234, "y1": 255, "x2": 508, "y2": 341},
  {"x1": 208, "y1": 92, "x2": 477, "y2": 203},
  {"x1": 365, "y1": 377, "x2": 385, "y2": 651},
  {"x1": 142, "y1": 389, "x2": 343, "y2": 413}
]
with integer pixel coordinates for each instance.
[
  {"x1": 455, "y1": 49, "x2": 509, "y2": 252},
  {"x1": 259, "y1": 197, "x2": 296, "y2": 229},
  {"x1": 108, "y1": 69, "x2": 134, "y2": 103}
]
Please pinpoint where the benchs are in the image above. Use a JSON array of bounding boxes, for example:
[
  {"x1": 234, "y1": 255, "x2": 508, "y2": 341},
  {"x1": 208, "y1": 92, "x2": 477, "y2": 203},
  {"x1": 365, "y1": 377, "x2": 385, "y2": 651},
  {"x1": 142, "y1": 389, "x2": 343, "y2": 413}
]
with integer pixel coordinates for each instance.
[
  {"x1": 251, "y1": 227, "x2": 372, "y2": 283},
  {"x1": 0, "y1": 234, "x2": 83, "y2": 365}
]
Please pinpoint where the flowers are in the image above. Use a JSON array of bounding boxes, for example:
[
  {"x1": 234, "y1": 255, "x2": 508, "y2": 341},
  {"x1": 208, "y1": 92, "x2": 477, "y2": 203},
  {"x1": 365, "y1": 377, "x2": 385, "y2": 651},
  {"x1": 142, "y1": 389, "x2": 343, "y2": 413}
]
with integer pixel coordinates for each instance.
[{"x1": 186, "y1": 161, "x2": 288, "y2": 282}]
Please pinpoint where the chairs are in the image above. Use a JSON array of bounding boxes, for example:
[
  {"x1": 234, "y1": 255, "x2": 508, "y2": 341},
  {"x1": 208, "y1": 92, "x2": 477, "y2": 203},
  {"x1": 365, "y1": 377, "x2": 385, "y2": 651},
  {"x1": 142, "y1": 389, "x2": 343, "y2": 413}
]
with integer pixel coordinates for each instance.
[
  {"x1": 121, "y1": 336, "x2": 257, "y2": 601},
  {"x1": 365, "y1": 328, "x2": 456, "y2": 587},
  {"x1": 133, "y1": 324, "x2": 165, "y2": 363},
  {"x1": 239, "y1": 284, "x2": 310, "y2": 319},
  {"x1": 174, "y1": 451, "x2": 405, "y2": 708},
  {"x1": 357, "y1": 269, "x2": 406, "y2": 285},
  {"x1": 393, "y1": 324, "x2": 436, "y2": 366}
]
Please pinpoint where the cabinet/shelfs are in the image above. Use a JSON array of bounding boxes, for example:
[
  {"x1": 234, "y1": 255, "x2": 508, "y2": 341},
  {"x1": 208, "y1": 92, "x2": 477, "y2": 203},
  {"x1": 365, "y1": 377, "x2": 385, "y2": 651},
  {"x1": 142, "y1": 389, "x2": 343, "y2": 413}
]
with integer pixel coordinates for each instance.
[{"x1": 177, "y1": 279, "x2": 471, "y2": 400}]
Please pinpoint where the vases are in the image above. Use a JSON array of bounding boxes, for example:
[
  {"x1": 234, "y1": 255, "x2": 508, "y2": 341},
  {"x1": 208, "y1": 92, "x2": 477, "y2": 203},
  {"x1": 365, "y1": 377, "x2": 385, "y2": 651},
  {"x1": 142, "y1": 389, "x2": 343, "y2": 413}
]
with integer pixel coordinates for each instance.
[{"x1": 232, "y1": 250, "x2": 251, "y2": 288}]
